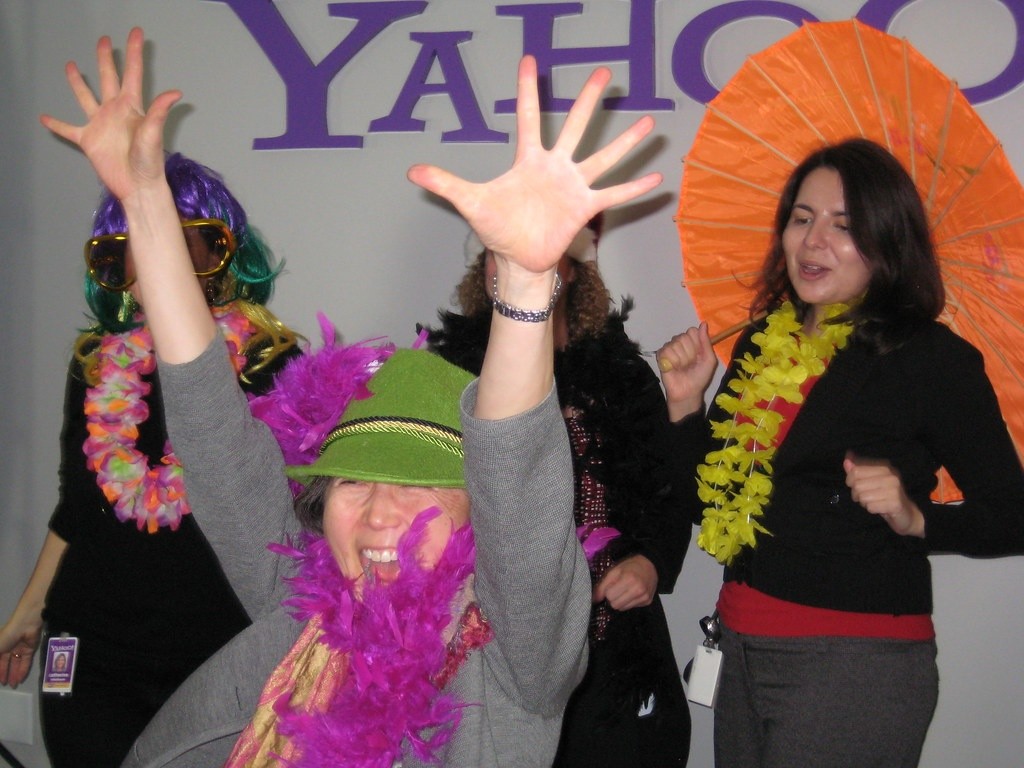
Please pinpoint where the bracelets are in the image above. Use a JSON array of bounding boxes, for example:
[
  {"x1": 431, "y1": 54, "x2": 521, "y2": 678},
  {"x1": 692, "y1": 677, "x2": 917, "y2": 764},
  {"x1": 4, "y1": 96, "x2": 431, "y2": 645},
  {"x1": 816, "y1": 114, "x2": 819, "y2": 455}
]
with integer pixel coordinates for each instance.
[{"x1": 491, "y1": 272, "x2": 563, "y2": 321}]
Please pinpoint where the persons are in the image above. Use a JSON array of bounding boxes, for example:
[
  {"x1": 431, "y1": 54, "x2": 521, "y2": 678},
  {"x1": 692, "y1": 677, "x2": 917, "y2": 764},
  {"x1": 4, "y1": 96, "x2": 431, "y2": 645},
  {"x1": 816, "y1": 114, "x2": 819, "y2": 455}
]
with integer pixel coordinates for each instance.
[
  {"x1": 0, "y1": 155, "x2": 303, "y2": 768},
  {"x1": 656, "y1": 141, "x2": 1024, "y2": 768},
  {"x1": 39, "y1": 27, "x2": 665, "y2": 768},
  {"x1": 54, "y1": 653, "x2": 66, "y2": 671},
  {"x1": 417, "y1": 213, "x2": 692, "y2": 768}
]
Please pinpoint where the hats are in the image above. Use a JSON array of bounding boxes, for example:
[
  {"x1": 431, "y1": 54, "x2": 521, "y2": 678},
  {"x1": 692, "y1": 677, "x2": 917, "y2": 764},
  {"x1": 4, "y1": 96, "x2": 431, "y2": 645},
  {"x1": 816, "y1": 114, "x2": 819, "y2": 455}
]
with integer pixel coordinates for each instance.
[
  {"x1": 249, "y1": 311, "x2": 478, "y2": 487},
  {"x1": 464, "y1": 224, "x2": 597, "y2": 267}
]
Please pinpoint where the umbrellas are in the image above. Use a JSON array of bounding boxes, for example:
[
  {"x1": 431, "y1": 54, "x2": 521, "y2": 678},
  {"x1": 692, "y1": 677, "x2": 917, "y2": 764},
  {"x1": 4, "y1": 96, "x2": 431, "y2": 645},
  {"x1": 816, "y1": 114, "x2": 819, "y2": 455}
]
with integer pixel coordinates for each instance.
[{"x1": 673, "y1": 20, "x2": 1024, "y2": 503}]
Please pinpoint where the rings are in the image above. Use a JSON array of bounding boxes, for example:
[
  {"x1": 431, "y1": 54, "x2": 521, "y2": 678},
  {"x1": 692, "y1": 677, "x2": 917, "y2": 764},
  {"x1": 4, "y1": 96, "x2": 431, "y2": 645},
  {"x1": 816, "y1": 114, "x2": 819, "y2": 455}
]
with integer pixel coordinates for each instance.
[{"x1": 14, "y1": 653, "x2": 20, "y2": 657}]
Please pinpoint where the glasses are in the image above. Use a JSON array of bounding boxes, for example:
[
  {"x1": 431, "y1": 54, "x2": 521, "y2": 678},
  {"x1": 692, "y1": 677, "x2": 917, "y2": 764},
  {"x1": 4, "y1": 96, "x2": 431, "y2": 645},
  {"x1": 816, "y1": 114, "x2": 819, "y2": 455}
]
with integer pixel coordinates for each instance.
[{"x1": 84, "y1": 219, "x2": 236, "y2": 291}]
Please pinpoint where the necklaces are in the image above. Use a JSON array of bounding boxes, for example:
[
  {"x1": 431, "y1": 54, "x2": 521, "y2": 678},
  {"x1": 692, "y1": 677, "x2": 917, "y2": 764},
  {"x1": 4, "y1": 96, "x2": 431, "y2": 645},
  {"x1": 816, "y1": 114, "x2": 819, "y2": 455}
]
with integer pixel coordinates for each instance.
[
  {"x1": 81, "y1": 303, "x2": 250, "y2": 533},
  {"x1": 696, "y1": 298, "x2": 854, "y2": 567}
]
